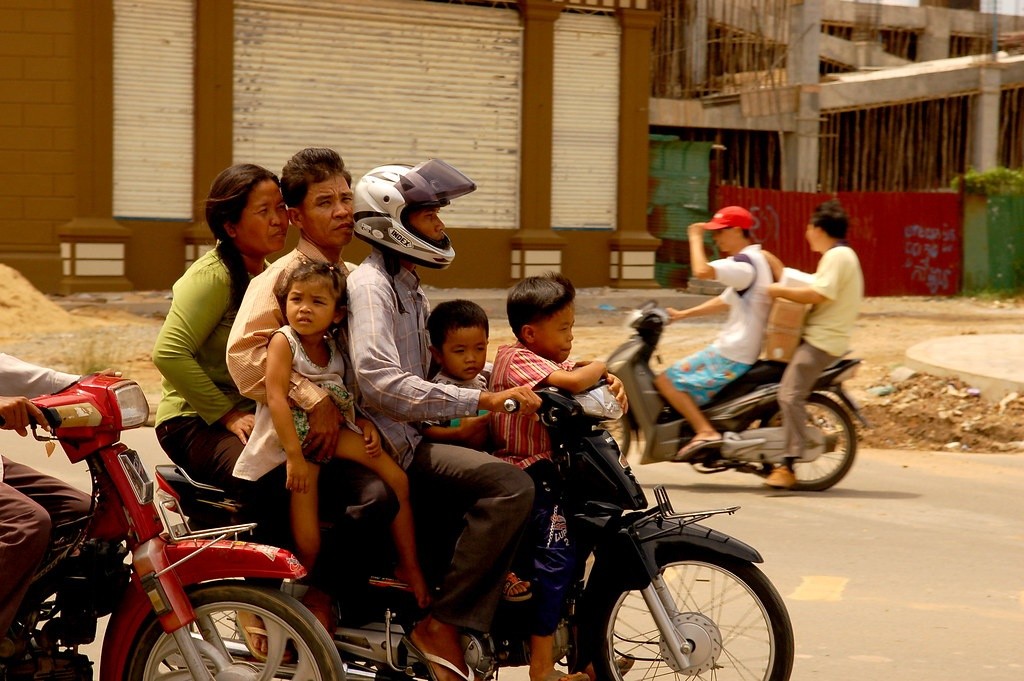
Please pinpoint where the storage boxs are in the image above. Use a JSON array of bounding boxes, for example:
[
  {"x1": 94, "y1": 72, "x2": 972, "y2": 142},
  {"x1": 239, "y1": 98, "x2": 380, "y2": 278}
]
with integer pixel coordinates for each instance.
[{"x1": 757, "y1": 296, "x2": 814, "y2": 364}]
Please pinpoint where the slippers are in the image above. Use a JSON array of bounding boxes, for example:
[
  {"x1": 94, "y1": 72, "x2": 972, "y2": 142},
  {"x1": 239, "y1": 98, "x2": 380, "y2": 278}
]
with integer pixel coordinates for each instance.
[
  {"x1": 236, "y1": 609, "x2": 291, "y2": 663},
  {"x1": 400, "y1": 634, "x2": 475, "y2": 681},
  {"x1": 615, "y1": 654, "x2": 634, "y2": 676},
  {"x1": 534, "y1": 671, "x2": 589, "y2": 681},
  {"x1": 501, "y1": 570, "x2": 532, "y2": 601},
  {"x1": 675, "y1": 439, "x2": 724, "y2": 460}
]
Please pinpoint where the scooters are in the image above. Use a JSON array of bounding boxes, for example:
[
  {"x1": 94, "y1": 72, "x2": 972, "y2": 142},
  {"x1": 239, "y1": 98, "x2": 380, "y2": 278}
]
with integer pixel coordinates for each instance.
[
  {"x1": 602, "y1": 300, "x2": 873, "y2": 491},
  {"x1": 156, "y1": 376, "x2": 794, "y2": 681},
  {"x1": 0, "y1": 372, "x2": 345, "y2": 681}
]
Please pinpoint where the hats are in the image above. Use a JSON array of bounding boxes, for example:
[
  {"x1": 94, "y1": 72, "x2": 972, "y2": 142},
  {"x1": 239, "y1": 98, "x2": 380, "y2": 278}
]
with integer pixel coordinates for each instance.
[{"x1": 699, "y1": 206, "x2": 758, "y2": 231}]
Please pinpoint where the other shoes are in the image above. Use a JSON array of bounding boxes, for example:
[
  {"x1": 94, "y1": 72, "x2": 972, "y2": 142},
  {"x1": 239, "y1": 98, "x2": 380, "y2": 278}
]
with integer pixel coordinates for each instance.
[
  {"x1": 763, "y1": 466, "x2": 795, "y2": 487},
  {"x1": 304, "y1": 594, "x2": 335, "y2": 644}
]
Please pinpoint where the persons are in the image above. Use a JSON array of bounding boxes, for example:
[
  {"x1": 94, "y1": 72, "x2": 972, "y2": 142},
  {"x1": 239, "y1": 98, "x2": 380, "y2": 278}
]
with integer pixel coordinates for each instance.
[
  {"x1": 0, "y1": 354, "x2": 131, "y2": 681},
  {"x1": 652, "y1": 206, "x2": 774, "y2": 457},
  {"x1": 760, "y1": 200, "x2": 865, "y2": 490},
  {"x1": 152, "y1": 147, "x2": 634, "y2": 681}
]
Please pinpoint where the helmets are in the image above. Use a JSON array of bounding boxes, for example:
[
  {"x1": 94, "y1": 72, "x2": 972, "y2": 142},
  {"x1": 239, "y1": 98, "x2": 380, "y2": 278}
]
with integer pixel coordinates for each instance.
[{"x1": 346, "y1": 156, "x2": 478, "y2": 269}]
